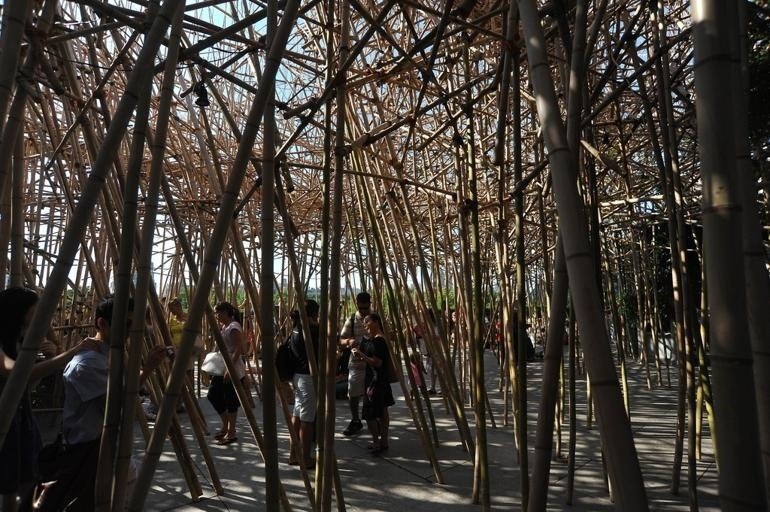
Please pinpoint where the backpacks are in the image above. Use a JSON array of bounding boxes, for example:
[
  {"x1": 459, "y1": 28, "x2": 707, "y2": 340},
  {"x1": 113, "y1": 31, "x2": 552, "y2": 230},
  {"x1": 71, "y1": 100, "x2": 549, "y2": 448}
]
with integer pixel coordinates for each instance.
[{"x1": 275, "y1": 330, "x2": 302, "y2": 382}]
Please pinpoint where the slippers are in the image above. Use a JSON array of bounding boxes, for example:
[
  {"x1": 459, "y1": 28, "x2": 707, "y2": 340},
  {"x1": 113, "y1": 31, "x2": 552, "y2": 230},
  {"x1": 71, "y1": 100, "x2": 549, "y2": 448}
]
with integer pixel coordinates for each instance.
[{"x1": 214, "y1": 430, "x2": 238, "y2": 445}]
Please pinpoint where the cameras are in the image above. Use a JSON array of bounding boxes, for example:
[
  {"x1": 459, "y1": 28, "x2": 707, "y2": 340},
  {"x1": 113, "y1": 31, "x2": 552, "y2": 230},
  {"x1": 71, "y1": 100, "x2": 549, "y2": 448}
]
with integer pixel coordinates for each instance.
[{"x1": 166, "y1": 346, "x2": 175, "y2": 359}]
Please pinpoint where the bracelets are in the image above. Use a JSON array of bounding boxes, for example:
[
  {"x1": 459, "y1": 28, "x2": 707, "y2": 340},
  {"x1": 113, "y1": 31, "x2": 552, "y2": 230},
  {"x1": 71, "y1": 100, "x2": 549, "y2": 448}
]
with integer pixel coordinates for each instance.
[{"x1": 347, "y1": 339, "x2": 350, "y2": 344}]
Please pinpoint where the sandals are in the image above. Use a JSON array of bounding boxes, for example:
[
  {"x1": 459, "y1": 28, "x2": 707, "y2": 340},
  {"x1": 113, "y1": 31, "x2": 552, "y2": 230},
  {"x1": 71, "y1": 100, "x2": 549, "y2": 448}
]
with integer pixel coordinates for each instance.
[{"x1": 366, "y1": 441, "x2": 390, "y2": 452}]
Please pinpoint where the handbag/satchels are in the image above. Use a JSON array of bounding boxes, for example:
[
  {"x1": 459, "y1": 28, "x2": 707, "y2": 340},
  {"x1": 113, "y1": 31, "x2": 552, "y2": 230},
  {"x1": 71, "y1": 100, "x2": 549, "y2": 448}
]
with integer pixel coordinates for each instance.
[
  {"x1": 200, "y1": 349, "x2": 227, "y2": 377},
  {"x1": 376, "y1": 361, "x2": 401, "y2": 384}
]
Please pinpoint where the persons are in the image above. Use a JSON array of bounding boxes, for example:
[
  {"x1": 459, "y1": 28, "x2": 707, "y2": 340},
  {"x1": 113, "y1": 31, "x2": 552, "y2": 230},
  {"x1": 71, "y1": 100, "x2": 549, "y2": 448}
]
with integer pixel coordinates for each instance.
[
  {"x1": 414, "y1": 322, "x2": 427, "y2": 354},
  {"x1": 206, "y1": 302, "x2": 243, "y2": 445},
  {"x1": 34, "y1": 295, "x2": 169, "y2": 511},
  {"x1": 426, "y1": 309, "x2": 442, "y2": 394},
  {"x1": 409, "y1": 352, "x2": 428, "y2": 400},
  {"x1": 340, "y1": 292, "x2": 375, "y2": 435},
  {"x1": 483, "y1": 309, "x2": 500, "y2": 350},
  {"x1": 291, "y1": 299, "x2": 320, "y2": 466},
  {"x1": 165, "y1": 298, "x2": 195, "y2": 412},
  {"x1": 351, "y1": 314, "x2": 395, "y2": 452},
  {"x1": 2, "y1": 286, "x2": 102, "y2": 511}
]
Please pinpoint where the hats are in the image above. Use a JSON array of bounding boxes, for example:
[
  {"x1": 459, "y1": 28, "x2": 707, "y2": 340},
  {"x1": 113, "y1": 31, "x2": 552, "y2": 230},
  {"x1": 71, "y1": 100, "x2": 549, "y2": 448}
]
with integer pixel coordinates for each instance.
[{"x1": 356, "y1": 293, "x2": 372, "y2": 304}]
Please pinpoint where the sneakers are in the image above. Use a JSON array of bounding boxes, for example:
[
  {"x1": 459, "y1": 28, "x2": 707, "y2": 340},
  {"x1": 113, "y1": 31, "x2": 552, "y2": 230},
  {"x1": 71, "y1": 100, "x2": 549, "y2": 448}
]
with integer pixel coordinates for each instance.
[
  {"x1": 343, "y1": 418, "x2": 363, "y2": 435},
  {"x1": 427, "y1": 389, "x2": 437, "y2": 395}
]
